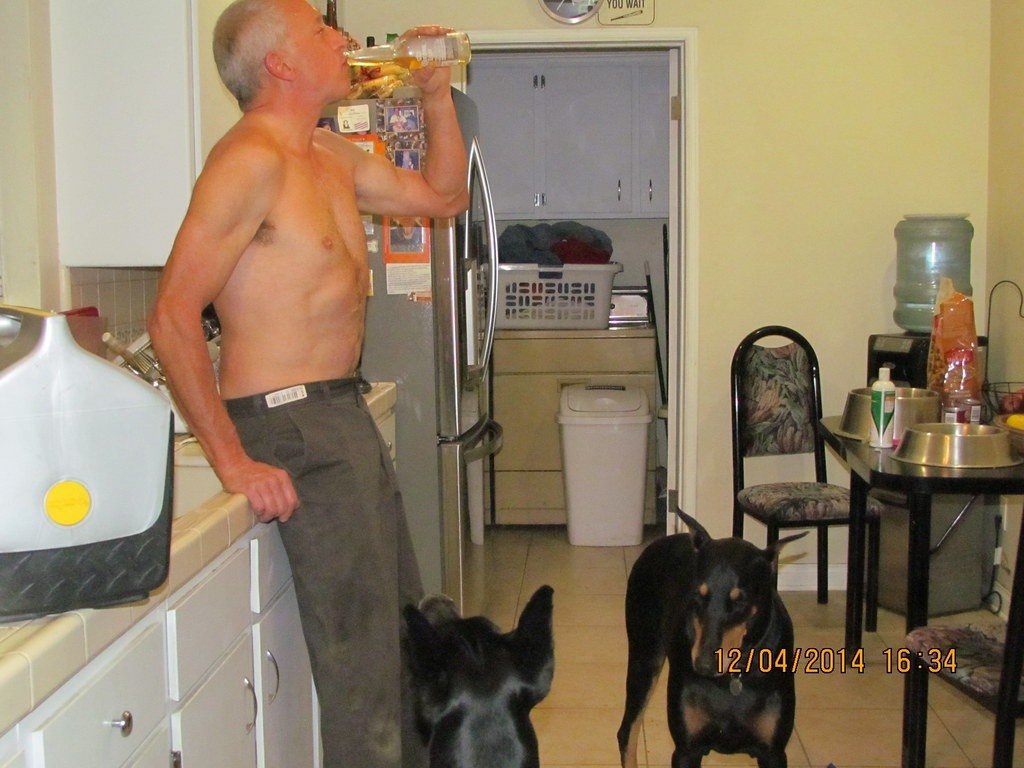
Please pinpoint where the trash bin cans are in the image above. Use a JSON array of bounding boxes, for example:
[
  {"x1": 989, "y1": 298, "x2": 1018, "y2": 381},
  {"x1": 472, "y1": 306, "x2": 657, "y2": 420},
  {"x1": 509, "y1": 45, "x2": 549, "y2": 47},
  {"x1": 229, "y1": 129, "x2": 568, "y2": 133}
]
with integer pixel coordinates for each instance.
[{"x1": 558, "y1": 381, "x2": 650, "y2": 547}]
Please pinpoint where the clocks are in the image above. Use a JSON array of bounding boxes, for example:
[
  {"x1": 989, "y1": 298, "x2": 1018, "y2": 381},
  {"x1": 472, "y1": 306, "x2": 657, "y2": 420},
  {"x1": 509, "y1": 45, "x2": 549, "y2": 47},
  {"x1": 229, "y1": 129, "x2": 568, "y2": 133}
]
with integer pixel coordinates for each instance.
[{"x1": 538, "y1": 0, "x2": 604, "y2": 25}]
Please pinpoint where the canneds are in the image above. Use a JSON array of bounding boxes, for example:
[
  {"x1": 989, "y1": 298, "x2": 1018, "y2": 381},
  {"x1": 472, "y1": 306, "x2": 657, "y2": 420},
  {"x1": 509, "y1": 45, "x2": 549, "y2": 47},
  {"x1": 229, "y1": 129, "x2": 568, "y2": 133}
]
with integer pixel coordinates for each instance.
[{"x1": 941, "y1": 399, "x2": 982, "y2": 423}]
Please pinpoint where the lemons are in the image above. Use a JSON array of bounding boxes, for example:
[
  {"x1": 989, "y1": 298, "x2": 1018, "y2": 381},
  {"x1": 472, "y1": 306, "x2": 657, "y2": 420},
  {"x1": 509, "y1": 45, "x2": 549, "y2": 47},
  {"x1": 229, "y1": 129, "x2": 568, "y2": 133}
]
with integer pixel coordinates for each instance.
[{"x1": 1007, "y1": 414, "x2": 1024, "y2": 430}]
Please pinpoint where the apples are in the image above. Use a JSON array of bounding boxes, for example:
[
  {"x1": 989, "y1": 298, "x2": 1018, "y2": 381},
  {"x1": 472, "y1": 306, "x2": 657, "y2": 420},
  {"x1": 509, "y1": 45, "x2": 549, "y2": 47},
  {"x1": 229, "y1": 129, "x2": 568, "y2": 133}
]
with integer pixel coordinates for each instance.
[{"x1": 1000, "y1": 388, "x2": 1024, "y2": 413}]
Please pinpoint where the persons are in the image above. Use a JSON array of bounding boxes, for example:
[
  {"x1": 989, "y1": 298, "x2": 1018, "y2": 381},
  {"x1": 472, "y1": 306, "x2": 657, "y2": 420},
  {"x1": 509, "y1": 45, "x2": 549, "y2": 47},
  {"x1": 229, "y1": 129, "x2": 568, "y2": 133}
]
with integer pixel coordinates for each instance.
[
  {"x1": 390, "y1": 216, "x2": 423, "y2": 252},
  {"x1": 145, "y1": 0, "x2": 470, "y2": 768},
  {"x1": 389, "y1": 108, "x2": 407, "y2": 131}
]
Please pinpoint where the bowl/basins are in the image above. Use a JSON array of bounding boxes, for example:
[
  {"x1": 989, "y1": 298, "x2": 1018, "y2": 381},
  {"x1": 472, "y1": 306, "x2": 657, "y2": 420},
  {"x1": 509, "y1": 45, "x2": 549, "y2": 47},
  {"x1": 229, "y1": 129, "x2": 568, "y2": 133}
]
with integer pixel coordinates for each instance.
[
  {"x1": 990, "y1": 412, "x2": 1024, "y2": 459},
  {"x1": 890, "y1": 423, "x2": 1024, "y2": 468},
  {"x1": 832, "y1": 387, "x2": 940, "y2": 442}
]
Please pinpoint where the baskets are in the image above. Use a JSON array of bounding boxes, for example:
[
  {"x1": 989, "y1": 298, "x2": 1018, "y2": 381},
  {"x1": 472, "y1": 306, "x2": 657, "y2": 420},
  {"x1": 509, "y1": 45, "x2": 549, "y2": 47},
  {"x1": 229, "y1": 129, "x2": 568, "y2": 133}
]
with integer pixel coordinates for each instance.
[{"x1": 482, "y1": 262, "x2": 625, "y2": 331}]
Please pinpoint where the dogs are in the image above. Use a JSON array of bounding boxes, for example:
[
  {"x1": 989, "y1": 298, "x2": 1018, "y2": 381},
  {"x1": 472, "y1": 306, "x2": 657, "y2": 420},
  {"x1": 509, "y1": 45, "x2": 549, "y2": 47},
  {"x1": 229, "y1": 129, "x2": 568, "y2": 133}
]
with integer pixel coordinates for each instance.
[
  {"x1": 403, "y1": 585, "x2": 555, "y2": 768},
  {"x1": 616, "y1": 507, "x2": 809, "y2": 768}
]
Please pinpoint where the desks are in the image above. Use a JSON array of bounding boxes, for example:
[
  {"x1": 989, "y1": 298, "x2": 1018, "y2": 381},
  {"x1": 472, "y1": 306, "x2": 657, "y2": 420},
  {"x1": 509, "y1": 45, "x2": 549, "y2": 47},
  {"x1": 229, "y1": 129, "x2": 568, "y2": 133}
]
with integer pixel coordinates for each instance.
[{"x1": 816, "y1": 415, "x2": 1024, "y2": 768}]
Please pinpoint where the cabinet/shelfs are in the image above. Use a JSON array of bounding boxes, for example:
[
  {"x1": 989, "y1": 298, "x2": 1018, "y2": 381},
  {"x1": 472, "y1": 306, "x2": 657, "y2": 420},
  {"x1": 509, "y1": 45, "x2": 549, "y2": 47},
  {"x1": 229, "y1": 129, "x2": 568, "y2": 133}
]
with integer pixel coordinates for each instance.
[
  {"x1": 465, "y1": 48, "x2": 672, "y2": 227},
  {"x1": 31, "y1": 619, "x2": 173, "y2": 768},
  {"x1": 169, "y1": 524, "x2": 318, "y2": 768},
  {"x1": 490, "y1": 328, "x2": 660, "y2": 529},
  {"x1": 310, "y1": 410, "x2": 399, "y2": 768},
  {"x1": 49, "y1": 0, "x2": 242, "y2": 264}
]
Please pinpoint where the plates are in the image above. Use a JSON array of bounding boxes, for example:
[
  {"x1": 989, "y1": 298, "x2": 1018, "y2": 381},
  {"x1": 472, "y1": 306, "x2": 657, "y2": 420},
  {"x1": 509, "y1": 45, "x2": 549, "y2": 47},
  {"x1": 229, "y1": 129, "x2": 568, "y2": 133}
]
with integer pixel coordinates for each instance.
[{"x1": 902, "y1": 214, "x2": 970, "y2": 220}]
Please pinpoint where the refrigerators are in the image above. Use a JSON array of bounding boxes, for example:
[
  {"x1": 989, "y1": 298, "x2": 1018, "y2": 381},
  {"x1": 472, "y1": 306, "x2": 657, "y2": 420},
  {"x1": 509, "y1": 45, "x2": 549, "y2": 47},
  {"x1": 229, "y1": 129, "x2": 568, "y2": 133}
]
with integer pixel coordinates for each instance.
[{"x1": 290, "y1": 84, "x2": 505, "y2": 623}]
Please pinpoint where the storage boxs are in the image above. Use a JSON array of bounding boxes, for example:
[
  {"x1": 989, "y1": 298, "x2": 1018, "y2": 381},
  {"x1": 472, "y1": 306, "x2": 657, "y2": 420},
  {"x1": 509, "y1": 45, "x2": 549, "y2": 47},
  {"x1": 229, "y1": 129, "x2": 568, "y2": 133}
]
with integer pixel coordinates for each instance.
[{"x1": 482, "y1": 260, "x2": 623, "y2": 327}]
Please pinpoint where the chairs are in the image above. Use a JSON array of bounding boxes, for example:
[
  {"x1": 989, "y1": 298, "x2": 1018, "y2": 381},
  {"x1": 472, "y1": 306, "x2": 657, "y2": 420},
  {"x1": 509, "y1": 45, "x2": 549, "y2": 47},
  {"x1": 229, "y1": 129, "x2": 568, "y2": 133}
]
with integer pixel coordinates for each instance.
[
  {"x1": 901, "y1": 494, "x2": 1024, "y2": 768},
  {"x1": 730, "y1": 326, "x2": 884, "y2": 635}
]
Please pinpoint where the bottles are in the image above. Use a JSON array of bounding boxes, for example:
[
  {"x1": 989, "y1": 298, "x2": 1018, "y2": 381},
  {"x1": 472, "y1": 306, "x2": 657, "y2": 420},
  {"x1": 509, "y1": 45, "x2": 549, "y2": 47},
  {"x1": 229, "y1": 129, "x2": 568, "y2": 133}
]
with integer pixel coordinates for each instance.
[
  {"x1": 869, "y1": 368, "x2": 896, "y2": 449},
  {"x1": 894, "y1": 220, "x2": 974, "y2": 335},
  {"x1": 343, "y1": 32, "x2": 472, "y2": 67}
]
